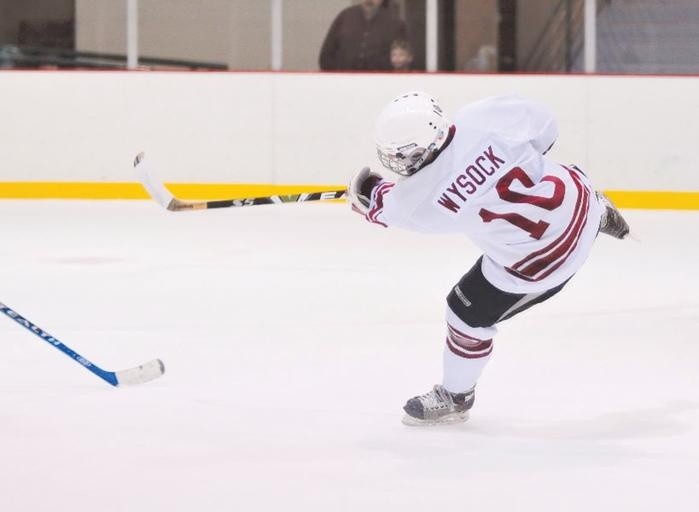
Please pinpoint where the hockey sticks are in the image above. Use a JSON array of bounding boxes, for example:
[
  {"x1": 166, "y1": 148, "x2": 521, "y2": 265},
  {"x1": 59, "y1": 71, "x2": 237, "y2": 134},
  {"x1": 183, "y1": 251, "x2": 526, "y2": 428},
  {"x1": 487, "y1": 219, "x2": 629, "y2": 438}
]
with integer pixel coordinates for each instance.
[
  {"x1": 134, "y1": 151, "x2": 345, "y2": 211},
  {"x1": 0, "y1": 304, "x2": 164, "y2": 388}
]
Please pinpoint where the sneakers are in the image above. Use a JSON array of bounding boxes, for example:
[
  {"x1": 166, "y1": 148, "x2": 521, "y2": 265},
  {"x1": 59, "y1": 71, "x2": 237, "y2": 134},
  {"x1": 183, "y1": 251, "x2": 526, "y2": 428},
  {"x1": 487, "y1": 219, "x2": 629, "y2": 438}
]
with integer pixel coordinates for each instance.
[
  {"x1": 405, "y1": 386, "x2": 475, "y2": 419},
  {"x1": 595, "y1": 192, "x2": 629, "y2": 240}
]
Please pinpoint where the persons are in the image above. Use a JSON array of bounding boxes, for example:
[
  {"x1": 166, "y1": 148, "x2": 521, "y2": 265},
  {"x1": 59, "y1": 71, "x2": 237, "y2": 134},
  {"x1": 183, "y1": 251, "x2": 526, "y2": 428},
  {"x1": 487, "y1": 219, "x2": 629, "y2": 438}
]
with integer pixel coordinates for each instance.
[
  {"x1": 387, "y1": 36, "x2": 422, "y2": 69},
  {"x1": 319, "y1": 0, "x2": 410, "y2": 69},
  {"x1": 346, "y1": 91, "x2": 626, "y2": 421}
]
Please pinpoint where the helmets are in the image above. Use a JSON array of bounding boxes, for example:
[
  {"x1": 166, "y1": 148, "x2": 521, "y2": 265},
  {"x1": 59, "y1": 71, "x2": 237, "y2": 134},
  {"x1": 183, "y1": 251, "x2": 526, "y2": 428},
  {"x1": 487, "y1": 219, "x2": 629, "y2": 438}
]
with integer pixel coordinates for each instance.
[{"x1": 377, "y1": 93, "x2": 449, "y2": 175}]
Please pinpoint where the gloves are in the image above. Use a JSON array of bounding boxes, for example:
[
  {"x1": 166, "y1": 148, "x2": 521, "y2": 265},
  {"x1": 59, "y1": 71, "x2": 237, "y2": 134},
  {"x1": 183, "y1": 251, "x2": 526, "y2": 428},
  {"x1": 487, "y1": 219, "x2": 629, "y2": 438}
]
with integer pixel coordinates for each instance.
[{"x1": 350, "y1": 166, "x2": 382, "y2": 214}]
